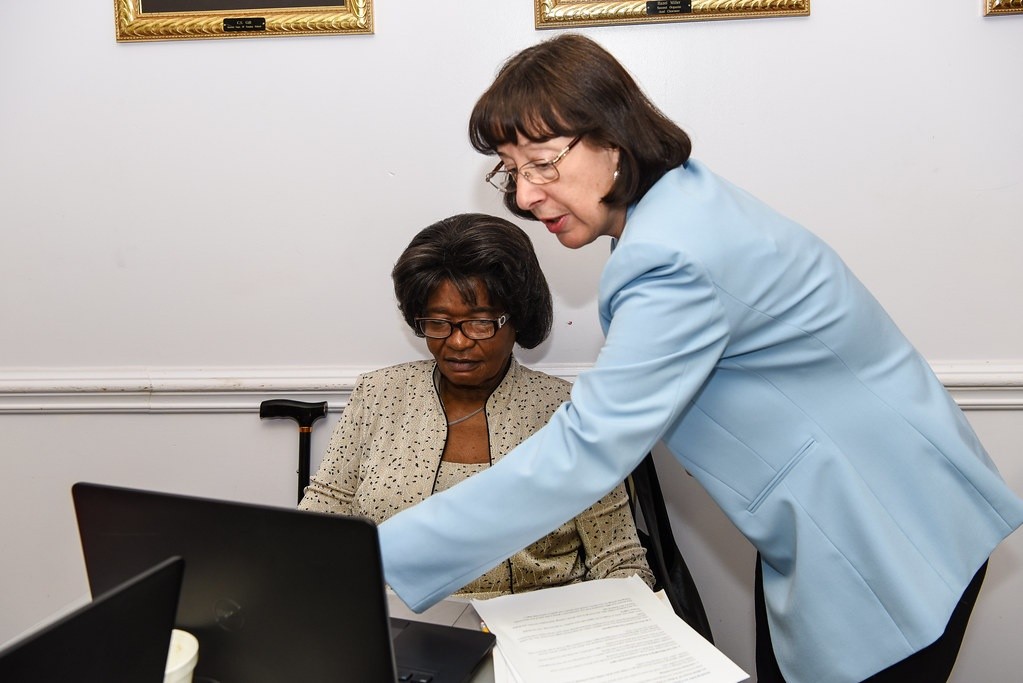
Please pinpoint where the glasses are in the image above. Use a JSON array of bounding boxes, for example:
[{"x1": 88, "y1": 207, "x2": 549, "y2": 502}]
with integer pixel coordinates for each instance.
[
  {"x1": 414, "y1": 311, "x2": 510, "y2": 340},
  {"x1": 485, "y1": 133, "x2": 584, "y2": 193}
]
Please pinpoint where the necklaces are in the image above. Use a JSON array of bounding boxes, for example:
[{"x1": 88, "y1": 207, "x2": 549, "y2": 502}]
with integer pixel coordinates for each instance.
[{"x1": 446, "y1": 399, "x2": 485, "y2": 427}]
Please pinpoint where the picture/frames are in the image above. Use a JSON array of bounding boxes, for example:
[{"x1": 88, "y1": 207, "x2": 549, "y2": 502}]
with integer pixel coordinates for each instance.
[
  {"x1": 113, "y1": 0, "x2": 375, "y2": 43},
  {"x1": 534, "y1": 0, "x2": 810, "y2": 30}
]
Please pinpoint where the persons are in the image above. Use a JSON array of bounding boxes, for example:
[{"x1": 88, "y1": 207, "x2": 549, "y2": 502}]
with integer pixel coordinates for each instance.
[
  {"x1": 290, "y1": 215, "x2": 660, "y2": 598},
  {"x1": 375, "y1": 31, "x2": 1021, "y2": 681}
]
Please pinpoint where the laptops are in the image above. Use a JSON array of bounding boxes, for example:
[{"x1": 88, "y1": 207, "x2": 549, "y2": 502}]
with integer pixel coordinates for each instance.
[
  {"x1": 0, "y1": 556, "x2": 185, "y2": 683},
  {"x1": 71, "y1": 481, "x2": 499, "y2": 683}
]
